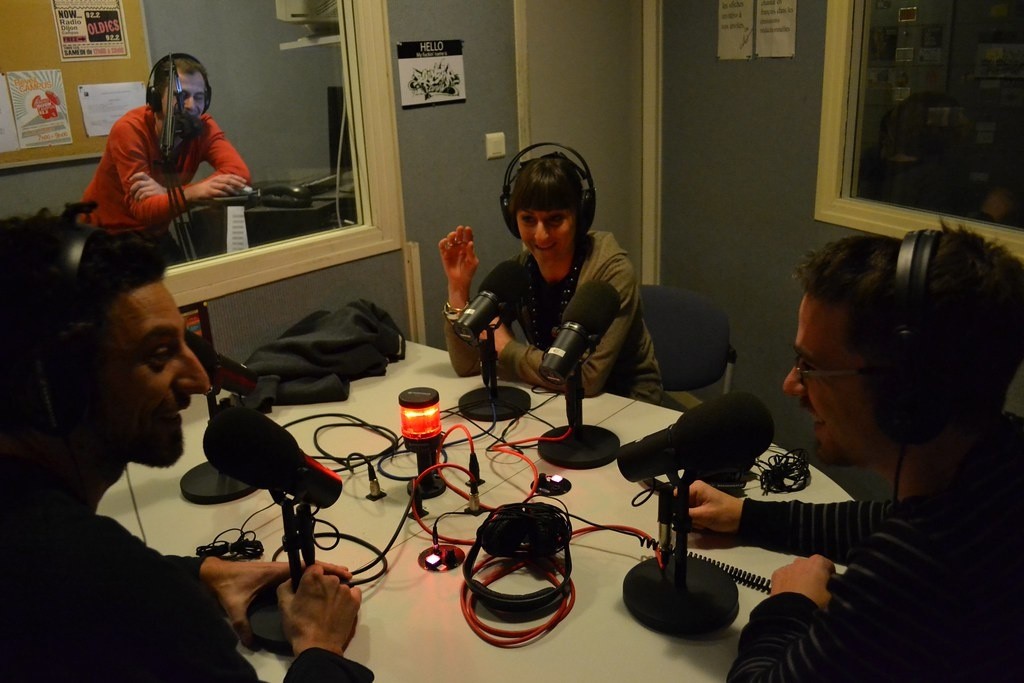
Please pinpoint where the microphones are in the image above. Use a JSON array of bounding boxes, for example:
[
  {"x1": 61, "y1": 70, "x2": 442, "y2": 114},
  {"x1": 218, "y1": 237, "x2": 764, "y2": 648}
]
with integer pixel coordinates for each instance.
[
  {"x1": 462, "y1": 502, "x2": 572, "y2": 623},
  {"x1": 539, "y1": 279, "x2": 621, "y2": 385},
  {"x1": 185, "y1": 329, "x2": 258, "y2": 397},
  {"x1": 452, "y1": 260, "x2": 528, "y2": 341},
  {"x1": 616, "y1": 391, "x2": 776, "y2": 482},
  {"x1": 203, "y1": 407, "x2": 342, "y2": 508},
  {"x1": 173, "y1": 92, "x2": 203, "y2": 139}
]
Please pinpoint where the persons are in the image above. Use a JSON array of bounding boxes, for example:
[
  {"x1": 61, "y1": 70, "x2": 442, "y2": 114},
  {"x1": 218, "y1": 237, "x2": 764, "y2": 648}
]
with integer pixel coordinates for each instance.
[
  {"x1": 0, "y1": 216, "x2": 374, "y2": 683},
  {"x1": 79, "y1": 53, "x2": 250, "y2": 266},
  {"x1": 439, "y1": 153, "x2": 664, "y2": 408},
  {"x1": 674, "y1": 215, "x2": 1024, "y2": 683}
]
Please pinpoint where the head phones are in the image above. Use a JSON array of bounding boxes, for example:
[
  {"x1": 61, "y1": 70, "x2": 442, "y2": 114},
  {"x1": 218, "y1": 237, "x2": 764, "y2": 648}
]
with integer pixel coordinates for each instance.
[
  {"x1": 500, "y1": 142, "x2": 597, "y2": 240},
  {"x1": 146, "y1": 52, "x2": 212, "y2": 115},
  {"x1": 16, "y1": 224, "x2": 114, "y2": 435},
  {"x1": 864, "y1": 228, "x2": 956, "y2": 445}
]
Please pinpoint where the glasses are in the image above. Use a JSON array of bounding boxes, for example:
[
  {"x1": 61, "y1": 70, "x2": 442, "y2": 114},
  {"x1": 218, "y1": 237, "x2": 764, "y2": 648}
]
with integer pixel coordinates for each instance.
[{"x1": 794, "y1": 358, "x2": 872, "y2": 382}]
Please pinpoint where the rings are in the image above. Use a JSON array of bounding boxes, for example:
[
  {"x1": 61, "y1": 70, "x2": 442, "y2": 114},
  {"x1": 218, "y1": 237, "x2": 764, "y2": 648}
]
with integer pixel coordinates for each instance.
[{"x1": 447, "y1": 241, "x2": 463, "y2": 247}]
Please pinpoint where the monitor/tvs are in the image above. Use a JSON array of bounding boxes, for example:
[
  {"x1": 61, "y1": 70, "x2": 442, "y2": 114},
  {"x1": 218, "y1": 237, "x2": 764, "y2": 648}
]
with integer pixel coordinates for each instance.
[
  {"x1": 276, "y1": 0, "x2": 339, "y2": 25},
  {"x1": 327, "y1": 86, "x2": 352, "y2": 175}
]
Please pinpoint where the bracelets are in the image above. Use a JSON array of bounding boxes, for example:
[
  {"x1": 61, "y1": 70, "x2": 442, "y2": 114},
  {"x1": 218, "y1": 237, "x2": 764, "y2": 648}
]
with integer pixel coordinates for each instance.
[{"x1": 446, "y1": 301, "x2": 468, "y2": 313}]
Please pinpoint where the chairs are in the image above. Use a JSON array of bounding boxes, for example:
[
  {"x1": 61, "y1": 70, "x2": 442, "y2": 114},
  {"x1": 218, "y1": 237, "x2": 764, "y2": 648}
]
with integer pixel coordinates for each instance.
[{"x1": 637, "y1": 284, "x2": 736, "y2": 413}]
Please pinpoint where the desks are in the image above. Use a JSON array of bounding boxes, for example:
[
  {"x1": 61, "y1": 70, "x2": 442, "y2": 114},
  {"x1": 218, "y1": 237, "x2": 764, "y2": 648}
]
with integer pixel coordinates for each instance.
[{"x1": 95, "y1": 338, "x2": 856, "y2": 683}]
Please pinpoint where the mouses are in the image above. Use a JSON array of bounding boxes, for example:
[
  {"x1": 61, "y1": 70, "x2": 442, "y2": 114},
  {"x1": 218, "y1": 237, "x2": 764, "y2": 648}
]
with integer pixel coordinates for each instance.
[{"x1": 234, "y1": 185, "x2": 254, "y2": 196}]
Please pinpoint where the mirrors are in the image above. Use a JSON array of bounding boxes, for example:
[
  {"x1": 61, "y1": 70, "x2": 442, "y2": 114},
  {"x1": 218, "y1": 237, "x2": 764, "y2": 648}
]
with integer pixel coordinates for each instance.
[
  {"x1": 1, "y1": 1, "x2": 363, "y2": 265},
  {"x1": 840, "y1": 0, "x2": 1024, "y2": 229}
]
